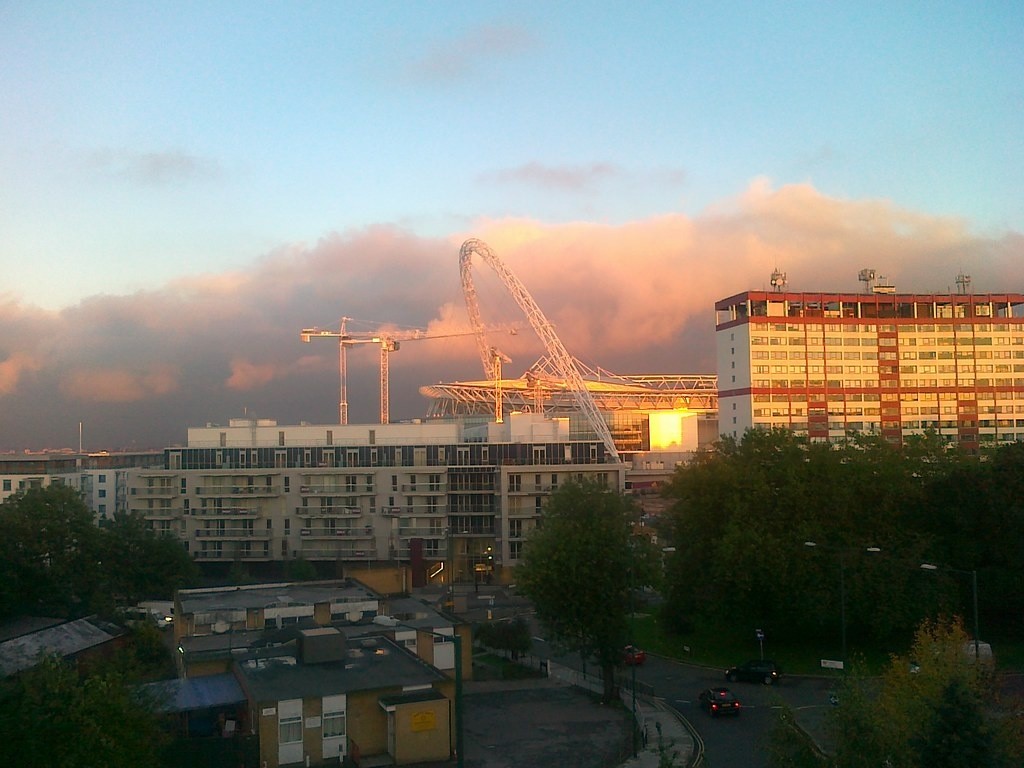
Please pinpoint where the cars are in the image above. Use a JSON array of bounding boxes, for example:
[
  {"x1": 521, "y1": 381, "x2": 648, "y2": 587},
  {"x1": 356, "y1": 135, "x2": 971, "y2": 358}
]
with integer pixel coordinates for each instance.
[
  {"x1": 699, "y1": 685, "x2": 741, "y2": 718},
  {"x1": 618, "y1": 644, "x2": 645, "y2": 666},
  {"x1": 725, "y1": 659, "x2": 783, "y2": 685},
  {"x1": 979, "y1": 670, "x2": 1024, "y2": 739}
]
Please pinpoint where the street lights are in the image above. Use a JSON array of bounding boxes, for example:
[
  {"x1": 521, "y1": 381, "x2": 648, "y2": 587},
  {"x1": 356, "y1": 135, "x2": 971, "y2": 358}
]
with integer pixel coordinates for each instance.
[
  {"x1": 920, "y1": 562, "x2": 980, "y2": 674},
  {"x1": 803, "y1": 541, "x2": 880, "y2": 676}
]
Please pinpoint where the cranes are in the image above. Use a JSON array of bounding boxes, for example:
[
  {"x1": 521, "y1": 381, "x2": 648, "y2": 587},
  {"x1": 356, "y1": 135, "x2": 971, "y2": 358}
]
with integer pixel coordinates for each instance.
[{"x1": 297, "y1": 313, "x2": 557, "y2": 424}]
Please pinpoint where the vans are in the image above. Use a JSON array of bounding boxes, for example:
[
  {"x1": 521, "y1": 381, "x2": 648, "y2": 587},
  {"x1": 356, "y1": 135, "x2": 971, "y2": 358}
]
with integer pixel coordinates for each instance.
[{"x1": 911, "y1": 639, "x2": 991, "y2": 680}]
